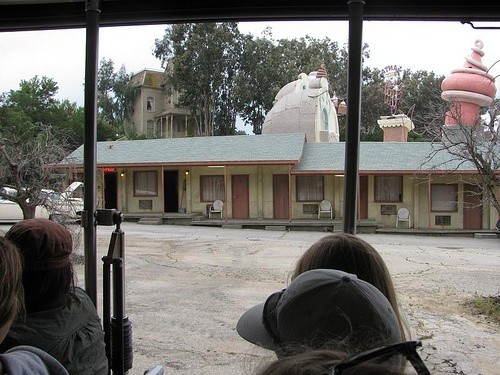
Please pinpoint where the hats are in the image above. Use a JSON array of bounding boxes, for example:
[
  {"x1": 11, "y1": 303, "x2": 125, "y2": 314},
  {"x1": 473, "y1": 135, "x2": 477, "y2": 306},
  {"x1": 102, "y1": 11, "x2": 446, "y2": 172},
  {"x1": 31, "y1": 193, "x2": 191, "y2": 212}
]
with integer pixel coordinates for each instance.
[
  {"x1": 236, "y1": 268, "x2": 404, "y2": 372},
  {"x1": 4, "y1": 218, "x2": 73, "y2": 271}
]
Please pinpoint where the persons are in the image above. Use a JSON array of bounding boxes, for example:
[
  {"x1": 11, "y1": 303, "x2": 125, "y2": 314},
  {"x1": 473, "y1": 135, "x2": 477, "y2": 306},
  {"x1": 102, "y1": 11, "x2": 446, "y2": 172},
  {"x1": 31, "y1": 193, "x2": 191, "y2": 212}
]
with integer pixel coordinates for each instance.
[
  {"x1": 0, "y1": 236, "x2": 68, "y2": 375},
  {"x1": 3, "y1": 217, "x2": 108, "y2": 375},
  {"x1": 235, "y1": 233, "x2": 408, "y2": 375}
]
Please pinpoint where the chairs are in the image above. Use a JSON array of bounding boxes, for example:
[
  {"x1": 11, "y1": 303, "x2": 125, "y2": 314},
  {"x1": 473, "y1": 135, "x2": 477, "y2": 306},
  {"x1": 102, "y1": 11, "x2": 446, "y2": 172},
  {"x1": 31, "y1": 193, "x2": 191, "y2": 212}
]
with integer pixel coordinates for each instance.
[
  {"x1": 395, "y1": 208, "x2": 412, "y2": 229},
  {"x1": 317, "y1": 199, "x2": 333, "y2": 221},
  {"x1": 208, "y1": 199, "x2": 223, "y2": 218}
]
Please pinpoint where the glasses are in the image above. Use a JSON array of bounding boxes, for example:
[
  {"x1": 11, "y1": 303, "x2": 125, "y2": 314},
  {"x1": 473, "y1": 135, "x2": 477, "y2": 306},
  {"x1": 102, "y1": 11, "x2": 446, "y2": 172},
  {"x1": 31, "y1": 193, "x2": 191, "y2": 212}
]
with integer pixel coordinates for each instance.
[
  {"x1": 263, "y1": 289, "x2": 288, "y2": 352},
  {"x1": 331, "y1": 340, "x2": 431, "y2": 375}
]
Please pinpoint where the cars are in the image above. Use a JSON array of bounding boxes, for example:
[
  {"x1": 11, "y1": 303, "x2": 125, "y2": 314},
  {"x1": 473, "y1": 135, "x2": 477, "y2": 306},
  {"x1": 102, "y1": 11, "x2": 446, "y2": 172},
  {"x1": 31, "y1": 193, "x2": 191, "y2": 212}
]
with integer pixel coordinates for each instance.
[{"x1": 0, "y1": 181, "x2": 85, "y2": 226}]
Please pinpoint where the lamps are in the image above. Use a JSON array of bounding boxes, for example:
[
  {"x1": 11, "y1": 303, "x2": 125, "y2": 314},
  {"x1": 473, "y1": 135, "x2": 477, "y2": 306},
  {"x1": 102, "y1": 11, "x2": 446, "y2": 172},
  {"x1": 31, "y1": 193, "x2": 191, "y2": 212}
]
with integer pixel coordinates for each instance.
[
  {"x1": 185, "y1": 168, "x2": 189, "y2": 175},
  {"x1": 121, "y1": 171, "x2": 125, "y2": 177}
]
